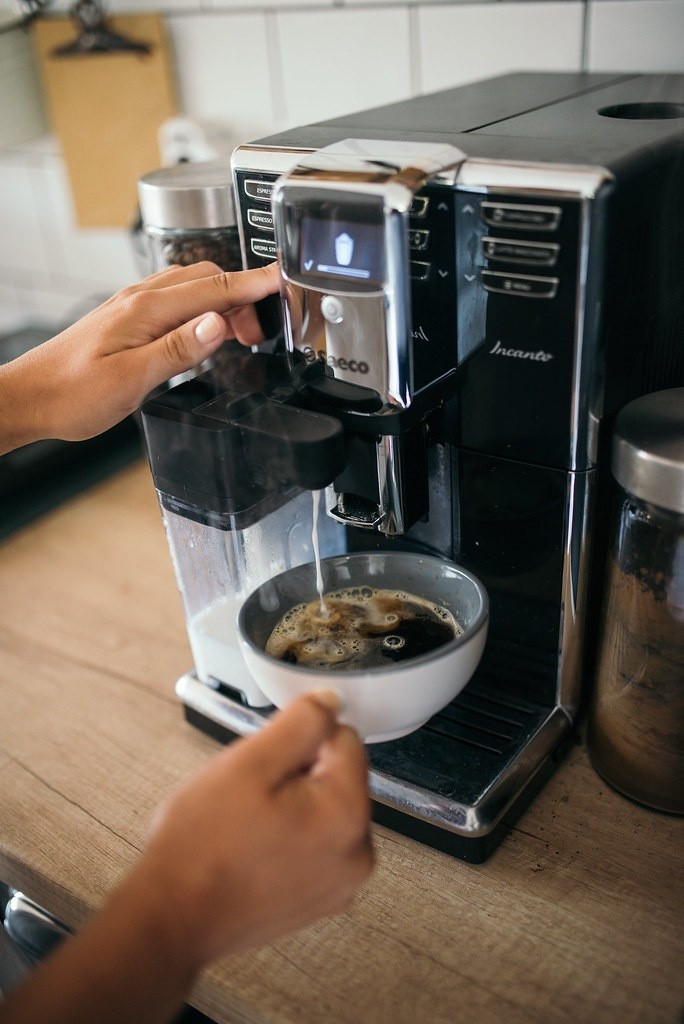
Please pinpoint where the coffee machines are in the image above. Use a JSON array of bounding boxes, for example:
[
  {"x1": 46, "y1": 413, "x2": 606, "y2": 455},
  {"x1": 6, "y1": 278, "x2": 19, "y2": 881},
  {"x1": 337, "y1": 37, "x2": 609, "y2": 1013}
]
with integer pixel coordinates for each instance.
[{"x1": 134, "y1": 70, "x2": 684, "y2": 906}]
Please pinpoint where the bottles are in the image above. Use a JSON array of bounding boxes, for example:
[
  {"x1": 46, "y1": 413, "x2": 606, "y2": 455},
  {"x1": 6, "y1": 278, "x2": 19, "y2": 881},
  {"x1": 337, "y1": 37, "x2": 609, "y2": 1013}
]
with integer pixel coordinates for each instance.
[
  {"x1": 586, "y1": 388, "x2": 682, "y2": 818},
  {"x1": 135, "y1": 169, "x2": 235, "y2": 315}
]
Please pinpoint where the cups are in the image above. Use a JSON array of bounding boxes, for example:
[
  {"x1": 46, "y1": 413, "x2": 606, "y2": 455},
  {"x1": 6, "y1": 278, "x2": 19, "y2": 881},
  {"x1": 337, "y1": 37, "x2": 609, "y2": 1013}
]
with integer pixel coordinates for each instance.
[{"x1": 232, "y1": 549, "x2": 489, "y2": 743}]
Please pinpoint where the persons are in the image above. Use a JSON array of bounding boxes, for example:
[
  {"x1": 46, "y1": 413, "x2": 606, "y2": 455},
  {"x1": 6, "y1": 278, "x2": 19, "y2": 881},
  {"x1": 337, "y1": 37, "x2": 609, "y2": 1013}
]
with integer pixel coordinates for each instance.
[{"x1": 0, "y1": 260, "x2": 376, "y2": 1024}]
